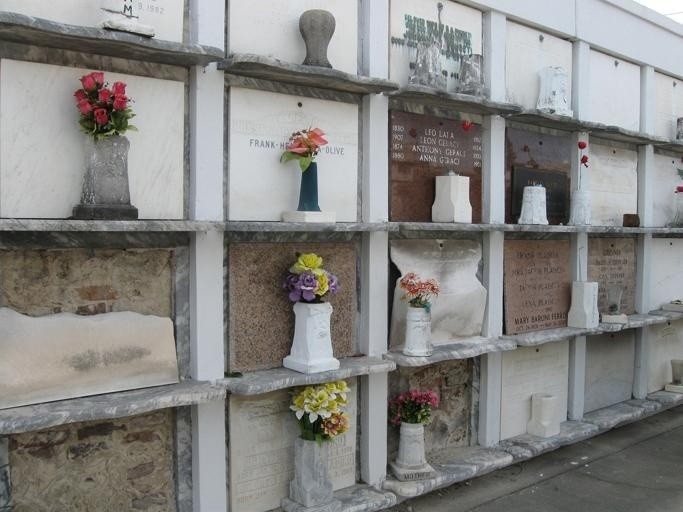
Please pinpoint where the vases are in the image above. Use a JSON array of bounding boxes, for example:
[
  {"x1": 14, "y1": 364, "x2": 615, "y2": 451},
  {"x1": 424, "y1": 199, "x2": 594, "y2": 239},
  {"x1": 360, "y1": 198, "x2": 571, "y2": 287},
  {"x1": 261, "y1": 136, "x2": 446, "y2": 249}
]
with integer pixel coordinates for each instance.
[
  {"x1": 524, "y1": 393, "x2": 561, "y2": 438},
  {"x1": 566, "y1": 280, "x2": 627, "y2": 330},
  {"x1": 568, "y1": 191, "x2": 592, "y2": 227},
  {"x1": 388, "y1": 425, "x2": 438, "y2": 481},
  {"x1": 72, "y1": 133, "x2": 138, "y2": 223},
  {"x1": 673, "y1": 194, "x2": 683, "y2": 228},
  {"x1": 428, "y1": 174, "x2": 552, "y2": 228},
  {"x1": 280, "y1": 436, "x2": 342, "y2": 512},
  {"x1": 534, "y1": 63, "x2": 577, "y2": 119},
  {"x1": 296, "y1": 8, "x2": 339, "y2": 69},
  {"x1": 405, "y1": 36, "x2": 491, "y2": 99},
  {"x1": 404, "y1": 310, "x2": 435, "y2": 358},
  {"x1": 674, "y1": 117, "x2": 682, "y2": 144},
  {"x1": 664, "y1": 359, "x2": 683, "y2": 394},
  {"x1": 282, "y1": 303, "x2": 341, "y2": 374},
  {"x1": 282, "y1": 163, "x2": 337, "y2": 225}
]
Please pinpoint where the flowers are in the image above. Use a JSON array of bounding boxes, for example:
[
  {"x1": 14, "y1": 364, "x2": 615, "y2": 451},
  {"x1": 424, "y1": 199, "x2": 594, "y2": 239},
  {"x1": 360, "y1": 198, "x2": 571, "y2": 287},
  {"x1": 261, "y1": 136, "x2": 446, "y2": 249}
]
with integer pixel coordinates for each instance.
[
  {"x1": 398, "y1": 270, "x2": 438, "y2": 307},
  {"x1": 675, "y1": 185, "x2": 682, "y2": 194},
  {"x1": 281, "y1": 129, "x2": 327, "y2": 163},
  {"x1": 388, "y1": 390, "x2": 439, "y2": 425},
  {"x1": 74, "y1": 71, "x2": 129, "y2": 138},
  {"x1": 575, "y1": 140, "x2": 591, "y2": 191},
  {"x1": 289, "y1": 254, "x2": 339, "y2": 303},
  {"x1": 285, "y1": 382, "x2": 349, "y2": 442}
]
